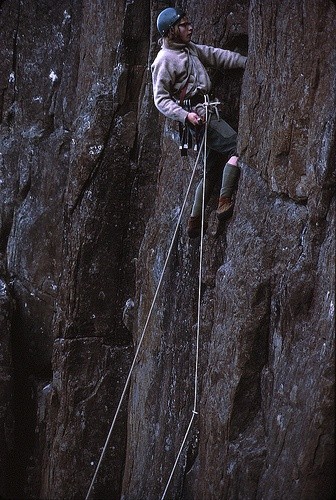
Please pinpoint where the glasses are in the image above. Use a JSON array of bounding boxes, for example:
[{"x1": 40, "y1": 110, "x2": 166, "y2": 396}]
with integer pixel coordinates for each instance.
[{"x1": 178, "y1": 21, "x2": 191, "y2": 26}]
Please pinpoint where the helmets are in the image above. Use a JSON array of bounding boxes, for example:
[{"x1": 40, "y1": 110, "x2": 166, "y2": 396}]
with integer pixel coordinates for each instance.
[{"x1": 156, "y1": 7, "x2": 186, "y2": 37}]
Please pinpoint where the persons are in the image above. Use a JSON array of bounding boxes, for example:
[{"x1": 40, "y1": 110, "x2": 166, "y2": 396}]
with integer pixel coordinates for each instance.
[{"x1": 151, "y1": 7, "x2": 248, "y2": 239}]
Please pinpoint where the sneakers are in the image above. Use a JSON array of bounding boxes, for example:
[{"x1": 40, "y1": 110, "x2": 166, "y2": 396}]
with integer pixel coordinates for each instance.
[
  {"x1": 186, "y1": 214, "x2": 208, "y2": 239},
  {"x1": 216, "y1": 196, "x2": 235, "y2": 217}
]
